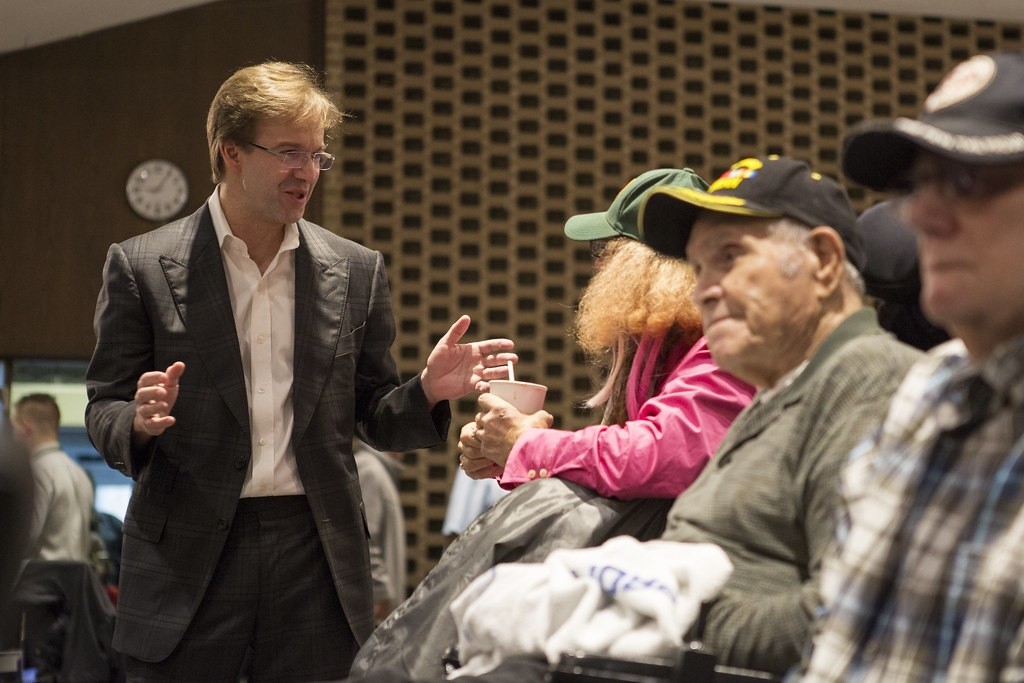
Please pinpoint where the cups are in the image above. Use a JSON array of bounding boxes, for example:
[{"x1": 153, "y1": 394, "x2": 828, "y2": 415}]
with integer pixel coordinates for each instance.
[{"x1": 488, "y1": 380, "x2": 547, "y2": 416}]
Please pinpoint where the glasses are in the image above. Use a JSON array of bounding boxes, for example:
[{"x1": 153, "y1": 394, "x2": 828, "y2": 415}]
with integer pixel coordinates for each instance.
[{"x1": 245, "y1": 142, "x2": 337, "y2": 171}]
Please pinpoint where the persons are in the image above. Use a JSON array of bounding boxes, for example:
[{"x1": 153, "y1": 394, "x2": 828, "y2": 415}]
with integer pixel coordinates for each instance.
[
  {"x1": 85, "y1": 62, "x2": 518, "y2": 683},
  {"x1": 782, "y1": 53, "x2": 1024, "y2": 683},
  {"x1": 444, "y1": 152, "x2": 960, "y2": 683},
  {"x1": 351, "y1": 437, "x2": 406, "y2": 628},
  {"x1": 0, "y1": 393, "x2": 124, "y2": 683}
]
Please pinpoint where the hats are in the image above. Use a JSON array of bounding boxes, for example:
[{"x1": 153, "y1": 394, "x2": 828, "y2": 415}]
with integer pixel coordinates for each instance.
[
  {"x1": 636, "y1": 153, "x2": 867, "y2": 273},
  {"x1": 839, "y1": 50, "x2": 1024, "y2": 193},
  {"x1": 563, "y1": 167, "x2": 709, "y2": 242}
]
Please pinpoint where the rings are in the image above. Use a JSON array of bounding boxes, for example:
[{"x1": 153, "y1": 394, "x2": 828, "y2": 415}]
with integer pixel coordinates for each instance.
[{"x1": 460, "y1": 461, "x2": 464, "y2": 470}]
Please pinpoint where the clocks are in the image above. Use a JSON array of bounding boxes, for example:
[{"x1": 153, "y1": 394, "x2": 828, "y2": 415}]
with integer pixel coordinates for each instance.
[{"x1": 121, "y1": 156, "x2": 189, "y2": 222}]
